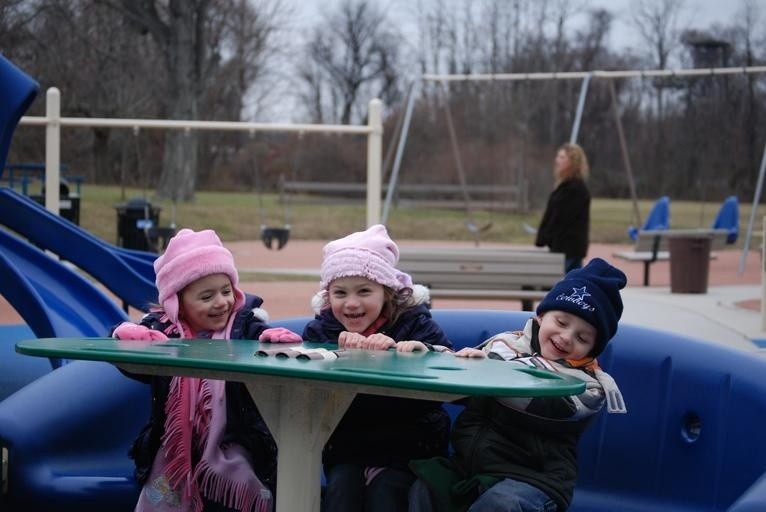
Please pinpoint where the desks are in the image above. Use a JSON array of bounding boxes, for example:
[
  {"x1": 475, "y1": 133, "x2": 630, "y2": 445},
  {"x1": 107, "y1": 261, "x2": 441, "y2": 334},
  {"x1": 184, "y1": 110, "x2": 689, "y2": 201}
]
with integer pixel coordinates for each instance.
[
  {"x1": 15, "y1": 333, "x2": 586, "y2": 512},
  {"x1": 641, "y1": 228, "x2": 729, "y2": 293}
]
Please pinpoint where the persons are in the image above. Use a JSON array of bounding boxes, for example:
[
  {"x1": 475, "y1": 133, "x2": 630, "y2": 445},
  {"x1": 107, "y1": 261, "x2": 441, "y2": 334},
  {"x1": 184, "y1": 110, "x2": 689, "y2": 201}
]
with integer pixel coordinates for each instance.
[
  {"x1": 521, "y1": 142, "x2": 592, "y2": 312},
  {"x1": 294, "y1": 222, "x2": 458, "y2": 511},
  {"x1": 379, "y1": 257, "x2": 628, "y2": 511},
  {"x1": 102, "y1": 226, "x2": 305, "y2": 511}
]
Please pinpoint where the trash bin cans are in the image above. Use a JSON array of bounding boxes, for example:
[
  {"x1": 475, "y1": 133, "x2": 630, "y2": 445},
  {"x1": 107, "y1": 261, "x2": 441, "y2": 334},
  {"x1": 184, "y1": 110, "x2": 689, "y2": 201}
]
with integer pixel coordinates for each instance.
[
  {"x1": 114, "y1": 197, "x2": 161, "y2": 253},
  {"x1": 669, "y1": 234, "x2": 712, "y2": 294},
  {"x1": 28, "y1": 178, "x2": 80, "y2": 226}
]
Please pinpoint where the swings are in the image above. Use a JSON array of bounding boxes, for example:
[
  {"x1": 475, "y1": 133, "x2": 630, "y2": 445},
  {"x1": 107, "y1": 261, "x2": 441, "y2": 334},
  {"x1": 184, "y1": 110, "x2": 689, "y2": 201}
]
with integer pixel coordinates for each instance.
[
  {"x1": 251, "y1": 130, "x2": 305, "y2": 251},
  {"x1": 703, "y1": 72, "x2": 753, "y2": 245},
  {"x1": 629, "y1": 78, "x2": 674, "y2": 240},
  {"x1": 134, "y1": 125, "x2": 189, "y2": 250},
  {"x1": 521, "y1": 76, "x2": 560, "y2": 232},
  {"x1": 443, "y1": 80, "x2": 495, "y2": 234}
]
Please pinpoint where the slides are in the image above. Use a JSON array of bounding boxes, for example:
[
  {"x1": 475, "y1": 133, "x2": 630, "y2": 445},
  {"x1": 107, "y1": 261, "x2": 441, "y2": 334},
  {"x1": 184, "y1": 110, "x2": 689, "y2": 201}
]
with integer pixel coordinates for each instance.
[{"x1": 0, "y1": 54, "x2": 161, "y2": 371}]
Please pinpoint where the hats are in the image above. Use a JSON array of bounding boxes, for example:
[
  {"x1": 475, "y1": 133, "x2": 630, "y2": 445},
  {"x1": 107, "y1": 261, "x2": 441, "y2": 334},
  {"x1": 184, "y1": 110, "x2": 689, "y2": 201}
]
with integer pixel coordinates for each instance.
[
  {"x1": 320, "y1": 223, "x2": 414, "y2": 293},
  {"x1": 536, "y1": 258, "x2": 627, "y2": 359},
  {"x1": 155, "y1": 228, "x2": 246, "y2": 326}
]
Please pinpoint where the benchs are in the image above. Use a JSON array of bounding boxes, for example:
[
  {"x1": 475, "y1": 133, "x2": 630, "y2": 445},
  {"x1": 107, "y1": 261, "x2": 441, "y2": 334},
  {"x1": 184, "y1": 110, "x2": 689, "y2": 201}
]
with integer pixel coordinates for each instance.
[
  {"x1": 392, "y1": 249, "x2": 566, "y2": 311},
  {"x1": 614, "y1": 250, "x2": 717, "y2": 287},
  {"x1": 0, "y1": 308, "x2": 766, "y2": 512}
]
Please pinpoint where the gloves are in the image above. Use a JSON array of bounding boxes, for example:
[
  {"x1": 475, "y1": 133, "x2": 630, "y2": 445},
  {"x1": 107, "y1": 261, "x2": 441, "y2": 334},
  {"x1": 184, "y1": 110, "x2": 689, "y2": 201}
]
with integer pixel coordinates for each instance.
[
  {"x1": 260, "y1": 327, "x2": 302, "y2": 343},
  {"x1": 113, "y1": 322, "x2": 169, "y2": 341}
]
